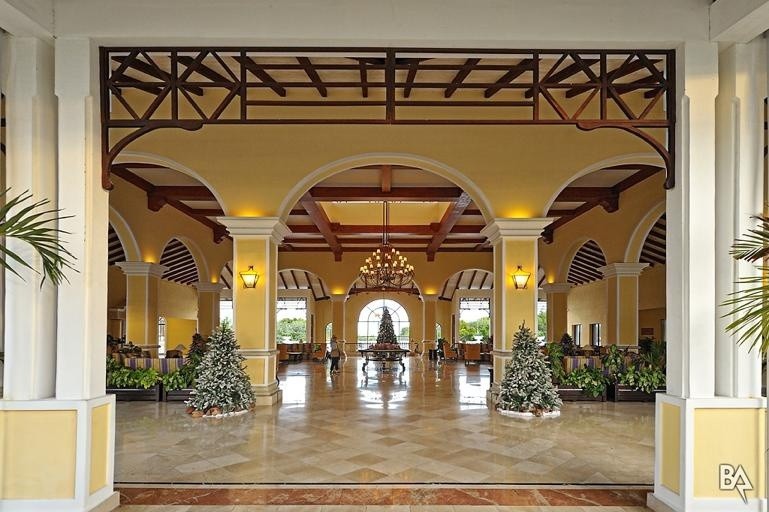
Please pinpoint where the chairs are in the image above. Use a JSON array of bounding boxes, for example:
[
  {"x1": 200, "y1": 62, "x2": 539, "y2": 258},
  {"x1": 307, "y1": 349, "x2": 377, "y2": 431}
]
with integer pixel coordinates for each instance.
[
  {"x1": 277, "y1": 343, "x2": 328, "y2": 364},
  {"x1": 442, "y1": 340, "x2": 493, "y2": 365}
]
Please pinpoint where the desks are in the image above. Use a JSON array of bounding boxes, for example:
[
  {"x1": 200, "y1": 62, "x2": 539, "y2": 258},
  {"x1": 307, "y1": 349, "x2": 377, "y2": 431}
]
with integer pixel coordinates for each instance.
[{"x1": 358, "y1": 350, "x2": 410, "y2": 372}]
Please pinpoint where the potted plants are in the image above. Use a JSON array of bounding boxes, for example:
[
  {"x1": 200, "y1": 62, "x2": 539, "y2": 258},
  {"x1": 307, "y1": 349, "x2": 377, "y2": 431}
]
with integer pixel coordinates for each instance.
[
  {"x1": 545, "y1": 341, "x2": 666, "y2": 402},
  {"x1": 106, "y1": 350, "x2": 202, "y2": 402}
]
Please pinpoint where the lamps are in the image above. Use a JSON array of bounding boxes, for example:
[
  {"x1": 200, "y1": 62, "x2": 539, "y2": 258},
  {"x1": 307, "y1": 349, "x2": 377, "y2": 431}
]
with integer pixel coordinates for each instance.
[
  {"x1": 239, "y1": 265, "x2": 260, "y2": 289},
  {"x1": 511, "y1": 265, "x2": 531, "y2": 290},
  {"x1": 358, "y1": 202, "x2": 417, "y2": 290}
]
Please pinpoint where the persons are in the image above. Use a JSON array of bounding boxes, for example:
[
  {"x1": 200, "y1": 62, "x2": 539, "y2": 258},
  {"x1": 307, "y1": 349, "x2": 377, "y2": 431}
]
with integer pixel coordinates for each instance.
[{"x1": 329, "y1": 334, "x2": 341, "y2": 373}]
